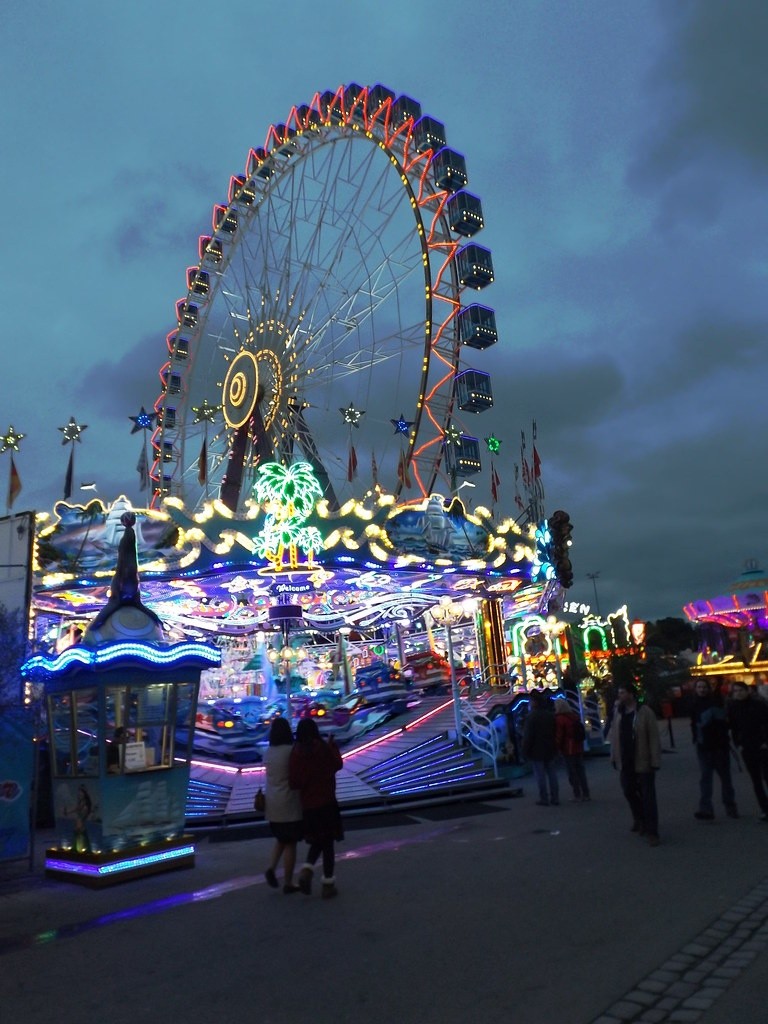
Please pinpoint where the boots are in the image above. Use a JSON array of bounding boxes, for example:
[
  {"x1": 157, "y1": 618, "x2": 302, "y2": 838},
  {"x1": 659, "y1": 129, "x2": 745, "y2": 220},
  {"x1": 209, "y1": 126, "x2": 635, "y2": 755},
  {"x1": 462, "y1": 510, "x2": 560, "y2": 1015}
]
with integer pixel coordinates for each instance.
[
  {"x1": 322, "y1": 877, "x2": 338, "y2": 900},
  {"x1": 299, "y1": 863, "x2": 314, "y2": 897}
]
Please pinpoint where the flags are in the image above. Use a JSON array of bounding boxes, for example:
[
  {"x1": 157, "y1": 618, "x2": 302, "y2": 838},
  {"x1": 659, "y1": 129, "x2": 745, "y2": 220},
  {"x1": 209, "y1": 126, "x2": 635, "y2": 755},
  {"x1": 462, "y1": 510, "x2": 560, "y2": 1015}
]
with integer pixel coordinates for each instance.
[
  {"x1": 63, "y1": 452, "x2": 72, "y2": 500},
  {"x1": 136, "y1": 444, "x2": 150, "y2": 491},
  {"x1": 197, "y1": 439, "x2": 206, "y2": 485},
  {"x1": 348, "y1": 442, "x2": 358, "y2": 482},
  {"x1": 372, "y1": 454, "x2": 377, "y2": 477},
  {"x1": 7, "y1": 459, "x2": 22, "y2": 510},
  {"x1": 491, "y1": 447, "x2": 541, "y2": 511},
  {"x1": 397, "y1": 450, "x2": 411, "y2": 488}
]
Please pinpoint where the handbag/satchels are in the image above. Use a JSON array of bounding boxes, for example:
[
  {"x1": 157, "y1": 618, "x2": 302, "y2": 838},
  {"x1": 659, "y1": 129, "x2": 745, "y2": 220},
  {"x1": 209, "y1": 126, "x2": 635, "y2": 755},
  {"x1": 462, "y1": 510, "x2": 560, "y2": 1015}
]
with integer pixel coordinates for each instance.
[{"x1": 253, "y1": 790, "x2": 266, "y2": 812}]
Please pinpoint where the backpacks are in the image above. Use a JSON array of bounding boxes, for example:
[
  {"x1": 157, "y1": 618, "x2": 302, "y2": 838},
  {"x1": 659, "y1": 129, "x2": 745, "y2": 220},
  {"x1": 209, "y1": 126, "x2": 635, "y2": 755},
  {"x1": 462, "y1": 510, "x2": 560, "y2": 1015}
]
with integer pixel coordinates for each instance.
[{"x1": 564, "y1": 714, "x2": 586, "y2": 743}]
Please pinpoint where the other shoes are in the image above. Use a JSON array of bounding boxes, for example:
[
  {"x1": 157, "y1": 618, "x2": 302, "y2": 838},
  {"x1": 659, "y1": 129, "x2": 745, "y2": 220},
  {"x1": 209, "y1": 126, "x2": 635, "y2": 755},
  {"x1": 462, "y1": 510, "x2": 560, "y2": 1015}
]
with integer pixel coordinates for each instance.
[
  {"x1": 265, "y1": 870, "x2": 279, "y2": 888},
  {"x1": 569, "y1": 795, "x2": 582, "y2": 803},
  {"x1": 727, "y1": 808, "x2": 738, "y2": 820},
  {"x1": 630, "y1": 818, "x2": 645, "y2": 835},
  {"x1": 694, "y1": 812, "x2": 714, "y2": 819},
  {"x1": 646, "y1": 833, "x2": 658, "y2": 847},
  {"x1": 536, "y1": 798, "x2": 549, "y2": 806},
  {"x1": 551, "y1": 798, "x2": 559, "y2": 806},
  {"x1": 283, "y1": 885, "x2": 299, "y2": 892}
]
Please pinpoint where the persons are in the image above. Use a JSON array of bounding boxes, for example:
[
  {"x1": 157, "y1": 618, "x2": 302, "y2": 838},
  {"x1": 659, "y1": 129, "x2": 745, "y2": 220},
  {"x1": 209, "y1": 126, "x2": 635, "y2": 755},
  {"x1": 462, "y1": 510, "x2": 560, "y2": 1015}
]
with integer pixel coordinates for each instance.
[
  {"x1": 264, "y1": 717, "x2": 343, "y2": 899},
  {"x1": 108, "y1": 727, "x2": 160, "y2": 775},
  {"x1": 519, "y1": 692, "x2": 590, "y2": 805},
  {"x1": 689, "y1": 679, "x2": 768, "y2": 821},
  {"x1": 610, "y1": 684, "x2": 661, "y2": 842}
]
